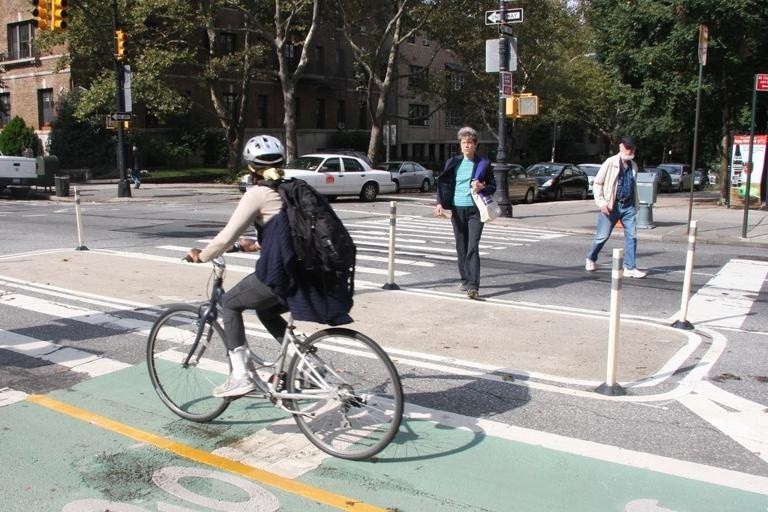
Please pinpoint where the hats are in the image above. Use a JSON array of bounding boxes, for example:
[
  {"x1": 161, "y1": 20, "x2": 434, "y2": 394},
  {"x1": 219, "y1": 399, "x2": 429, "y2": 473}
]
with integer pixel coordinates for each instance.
[{"x1": 618, "y1": 135, "x2": 636, "y2": 145}]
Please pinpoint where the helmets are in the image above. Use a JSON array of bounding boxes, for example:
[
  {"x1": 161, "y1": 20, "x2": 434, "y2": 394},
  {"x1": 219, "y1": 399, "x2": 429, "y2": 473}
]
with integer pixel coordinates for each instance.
[{"x1": 242, "y1": 135, "x2": 286, "y2": 164}]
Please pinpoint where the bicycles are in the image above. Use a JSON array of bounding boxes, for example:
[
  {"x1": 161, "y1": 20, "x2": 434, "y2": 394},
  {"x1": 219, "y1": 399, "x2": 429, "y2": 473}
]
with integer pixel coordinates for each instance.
[{"x1": 146, "y1": 244, "x2": 404, "y2": 461}]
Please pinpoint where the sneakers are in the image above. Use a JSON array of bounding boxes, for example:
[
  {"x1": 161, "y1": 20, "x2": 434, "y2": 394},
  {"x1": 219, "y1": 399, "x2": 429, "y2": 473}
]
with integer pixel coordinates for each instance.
[
  {"x1": 623, "y1": 267, "x2": 647, "y2": 279},
  {"x1": 585, "y1": 258, "x2": 596, "y2": 271},
  {"x1": 212, "y1": 372, "x2": 256, "y2": 397},
  {"x1": 299, "y1": 359, "x2": 327, "y2": 389}
]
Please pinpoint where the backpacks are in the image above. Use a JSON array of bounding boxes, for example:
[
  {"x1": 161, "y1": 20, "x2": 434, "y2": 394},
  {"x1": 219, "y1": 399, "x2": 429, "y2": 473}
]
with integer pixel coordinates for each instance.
[{"x1": 252, "y1": 177, "x2": 356, "y2": 272}]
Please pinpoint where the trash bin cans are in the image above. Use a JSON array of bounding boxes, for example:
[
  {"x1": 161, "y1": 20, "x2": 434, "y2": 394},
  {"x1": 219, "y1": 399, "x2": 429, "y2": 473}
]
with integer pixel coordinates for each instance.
[{"x1": 54, "y1": 173, "x2": 71, "y2": 197}]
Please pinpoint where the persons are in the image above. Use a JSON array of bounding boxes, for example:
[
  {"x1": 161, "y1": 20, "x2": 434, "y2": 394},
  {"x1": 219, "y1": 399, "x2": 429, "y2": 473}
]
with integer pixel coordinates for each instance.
[
  {"x1": 22, "y1": 147, "x2": 34, "y2": 158},
  {"x1": 433, "y1": 126, "x2": 497, "y2": 298},
  {"x1": 585, "y1": 135, "x2": 648, "y2": 280},
  {"x1": 128, "y1": 144, "x2": 149, "y2": 189},
  {"x1": 185, "y1": 134, "x2": 354, "y2": 398}
]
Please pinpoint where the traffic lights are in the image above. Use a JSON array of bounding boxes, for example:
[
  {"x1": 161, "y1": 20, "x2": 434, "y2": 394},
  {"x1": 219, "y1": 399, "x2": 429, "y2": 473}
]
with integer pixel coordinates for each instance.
[
  {"x1": 516, "y1": 94, "x2": 539, "y2": 117},
  {"x1": 51, "y1": 0, "x2": 68, "y2": 33},
  {"x1": 105, "y1": 114, "x2": 117, "y2": 130},
  {"x1": 116, "y1": 31, "x2": 128, "y2": 61},
  {"x1": 30, "y1": 0, "x2": 49, "y2": 30}
]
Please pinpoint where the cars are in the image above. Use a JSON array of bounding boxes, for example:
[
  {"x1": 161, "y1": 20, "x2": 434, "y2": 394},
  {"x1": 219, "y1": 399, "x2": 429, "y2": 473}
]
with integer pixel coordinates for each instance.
[
  {"x1": 485, "y1": 160, "x2": 718, "y2": 203},
  {"x1": 239, "y1": 150, "x2": 434, "y2": 203}
]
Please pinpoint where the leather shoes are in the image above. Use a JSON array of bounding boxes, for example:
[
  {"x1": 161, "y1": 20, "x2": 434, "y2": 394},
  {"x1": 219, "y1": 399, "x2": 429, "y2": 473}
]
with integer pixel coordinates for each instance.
[{"x1": 459, "y1": 284, "x2": 479, "y2": 297}]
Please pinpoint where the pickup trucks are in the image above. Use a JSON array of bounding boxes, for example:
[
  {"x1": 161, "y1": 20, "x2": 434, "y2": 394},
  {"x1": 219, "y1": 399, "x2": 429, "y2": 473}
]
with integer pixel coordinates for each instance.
[{"x1": 0, "y1": 148, "x2": 38, "y2": 197}]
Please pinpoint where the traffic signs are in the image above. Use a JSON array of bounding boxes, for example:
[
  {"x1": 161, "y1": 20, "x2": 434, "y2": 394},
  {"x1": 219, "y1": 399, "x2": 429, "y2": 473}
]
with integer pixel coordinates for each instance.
[
  {"x1": 497, "y1": 21, "x2": 514, "y2": 38},
  {"x1": 110, "y1": 112, "x2": 132, "y2": 121},
  {"x1": 484, "y1": 7, "x2": 523, "y2": 25}
]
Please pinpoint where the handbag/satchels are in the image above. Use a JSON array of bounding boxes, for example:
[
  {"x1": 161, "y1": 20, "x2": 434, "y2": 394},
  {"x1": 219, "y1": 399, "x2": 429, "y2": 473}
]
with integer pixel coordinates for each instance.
[{"x1": 470, "y1": 188, "x2": 502, "y2": 222}]
